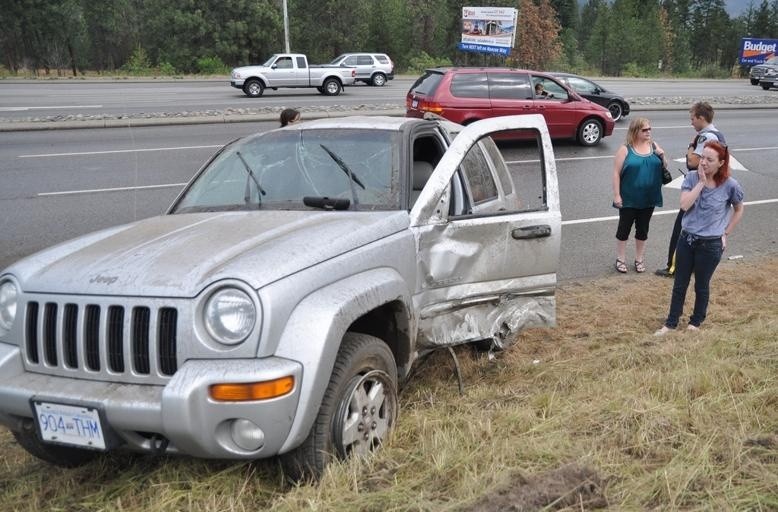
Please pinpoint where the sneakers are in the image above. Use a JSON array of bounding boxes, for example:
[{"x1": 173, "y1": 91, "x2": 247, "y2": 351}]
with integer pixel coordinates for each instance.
[
  {"x1": 654, "y1": 326, "x2": 676, "y2": 337},
  {"x1": 656, "y1": 268, "x2": 673, "y2": 276},
  {"x1": 686, "y1": 324, "x2": 696, "y2": 330}
]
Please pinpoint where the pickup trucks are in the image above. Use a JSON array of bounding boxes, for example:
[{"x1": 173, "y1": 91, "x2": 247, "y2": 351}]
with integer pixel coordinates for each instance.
[{"x1": 228, "y1": 52, "x2": 357, "y2": 94}]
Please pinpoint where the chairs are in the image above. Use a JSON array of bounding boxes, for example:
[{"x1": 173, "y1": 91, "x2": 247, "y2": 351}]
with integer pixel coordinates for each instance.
[{"x1": 410, "y1": 160, "x2": 433, "y2": 211}]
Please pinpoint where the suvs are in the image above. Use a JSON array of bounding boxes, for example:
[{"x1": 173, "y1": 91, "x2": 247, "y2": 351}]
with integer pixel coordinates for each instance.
[
  {"x1": 328, "y1": 53, "x2": 395, "y2": 86},
  {"x1": 748, "y1": 55, "x2": 778, "y2": 85},
  {"x1": 406, "y1": 67, "x2": 615, "y2": 147},
  {"x1": 0, "y1": 114, "x2": 564, "y2": 479}
]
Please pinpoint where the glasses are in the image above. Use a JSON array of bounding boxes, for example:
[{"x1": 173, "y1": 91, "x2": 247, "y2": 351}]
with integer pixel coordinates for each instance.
[
  {"x1": 711, "y1": 138, "x2": 728, "y2": 151},
  {"x1": 641, "y1": 128, "x2": 651, "y2": 132}
]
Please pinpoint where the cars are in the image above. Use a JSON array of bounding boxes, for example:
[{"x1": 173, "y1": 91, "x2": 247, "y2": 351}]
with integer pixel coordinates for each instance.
[
  {"x1": 541, "y1": 72, "x2": 631, "y2": 124},
  {"x1": 758, "y1": 65, "x2": 778, "y2": 91}
]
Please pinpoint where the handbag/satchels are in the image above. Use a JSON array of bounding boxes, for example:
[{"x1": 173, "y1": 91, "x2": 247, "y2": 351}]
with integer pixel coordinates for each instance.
[{"x1": 652, "y1": 143, "x2": 672, "y2": 185}]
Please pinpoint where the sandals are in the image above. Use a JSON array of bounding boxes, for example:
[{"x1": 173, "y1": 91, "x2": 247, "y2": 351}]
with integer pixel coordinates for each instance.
[
  {"x1": 616, "y1": 259, "x2": 627, "y2": 273},
  {"x1": 635, "y1": 260, "x2": 645, "y2": 273}
]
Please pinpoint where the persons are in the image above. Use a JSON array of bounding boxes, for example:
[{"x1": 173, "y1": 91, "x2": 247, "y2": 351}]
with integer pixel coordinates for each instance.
[
  {"x1": 535, "y1": 83, "x2": 551, "y2": 100},
  {"x1": 611, "y1": 116, "x2": 665, "y2": 275},
  {"x1": 656, "y1": 100, "x2": 728, "y2": 278},
  {"x1": 276, "y1": 108, "x2": 302, "y2": 131},
  {"x1": 649, "y1": 139, "x2": 745, "y2": 339}
]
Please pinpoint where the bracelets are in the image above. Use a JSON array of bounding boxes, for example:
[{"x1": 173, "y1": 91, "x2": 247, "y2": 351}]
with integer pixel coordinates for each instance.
[{"x1": 687, "y1": 142, "x2": 694, "y2": 150}]
